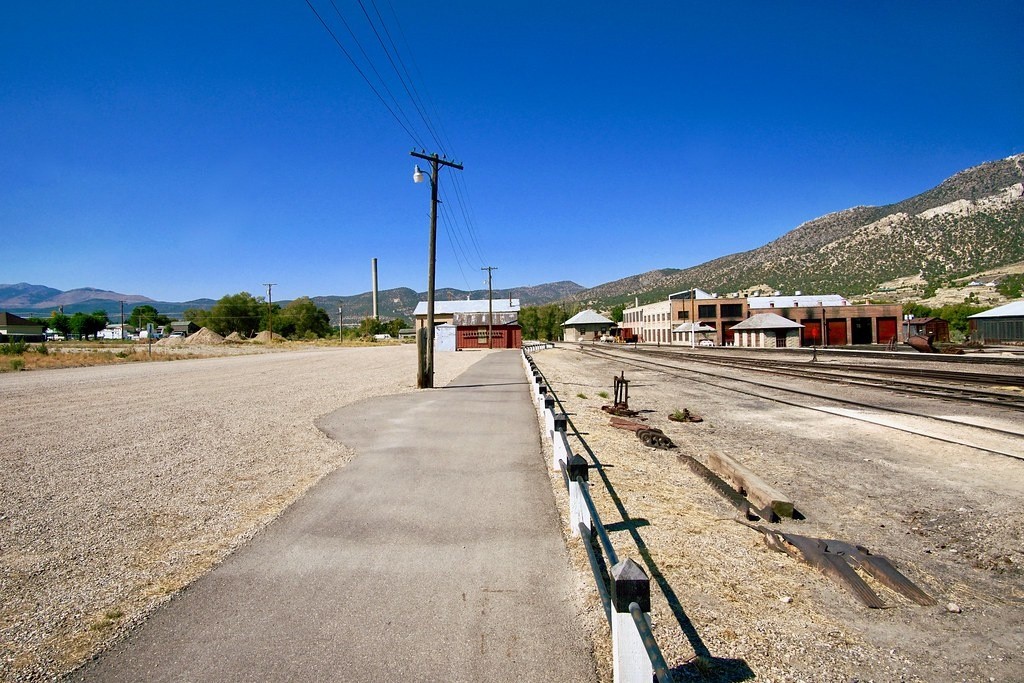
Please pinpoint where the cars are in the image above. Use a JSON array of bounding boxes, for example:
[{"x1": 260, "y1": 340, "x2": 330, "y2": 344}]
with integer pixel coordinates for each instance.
[{"x1": 169, "y1": 332, "x2": 185, "y2": 338}]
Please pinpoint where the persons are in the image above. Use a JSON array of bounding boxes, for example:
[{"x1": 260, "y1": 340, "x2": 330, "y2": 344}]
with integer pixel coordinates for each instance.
[
  {"x1": 964, "y1": 335, "x2": 969, "y2": 342},
  {"x1": 885, "y1": 335, "x2": 897, "y2": 352},
  {"x1": 918, "y1": 330, "x2": 923, "y2": 335},
  {"x1": 927, "y1": 331, "x2": 940, "y2": 352}
]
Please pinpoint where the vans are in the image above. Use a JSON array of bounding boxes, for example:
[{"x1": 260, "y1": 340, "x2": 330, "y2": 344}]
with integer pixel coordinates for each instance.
[{"x1": 373, "y1": 334, "x2": 391, "y2": 340}]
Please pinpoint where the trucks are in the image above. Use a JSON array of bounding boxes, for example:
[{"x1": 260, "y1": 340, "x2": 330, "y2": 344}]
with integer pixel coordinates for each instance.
[{"x1": 608, "y1": 328, "x2": 632, "y2": 343}]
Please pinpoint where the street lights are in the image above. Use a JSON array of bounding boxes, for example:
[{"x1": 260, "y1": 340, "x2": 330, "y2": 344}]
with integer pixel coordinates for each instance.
[
  {"x1": 904, "y1": 315, "x2": 914, "y2": 340},
  {"x1": 414, "y1": 165, "x2": 432, "y2": 185}
]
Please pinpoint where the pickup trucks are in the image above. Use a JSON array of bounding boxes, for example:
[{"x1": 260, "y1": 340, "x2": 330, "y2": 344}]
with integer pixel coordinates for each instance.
[
  {"x1": 47, "y1": 334, "x2": 65, "y2": 341},
  {"x1": 600, "y1": 334, "x2": 613, "y2": 343}
]
